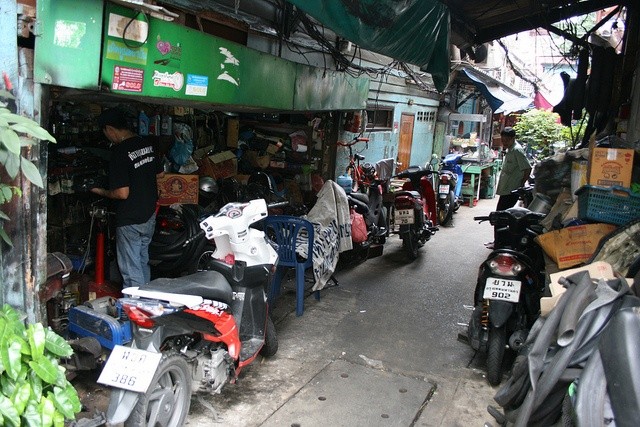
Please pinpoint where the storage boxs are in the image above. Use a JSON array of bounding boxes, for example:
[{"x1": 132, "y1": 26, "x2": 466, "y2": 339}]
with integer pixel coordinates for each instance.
[
  {"x1": 156, "y1": 174, "x2": 199, "y2": 206},
  {"x1": 533, "y1": 222, "x2": 618, "y2": 270},
  {"x1": 570, "y1": 159, "x2": 589, "y2": 203},
  {"x1": 586, "y1": 128, "x2": 635, "y2": 197},
  {"x1": 206, "y1": 150, "x2": 236, "y2": 180}
]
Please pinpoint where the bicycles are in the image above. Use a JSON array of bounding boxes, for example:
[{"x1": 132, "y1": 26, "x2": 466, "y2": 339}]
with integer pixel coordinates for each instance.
[{"x1": 331, "y1": 137, "x2": 378, "y2": 192}]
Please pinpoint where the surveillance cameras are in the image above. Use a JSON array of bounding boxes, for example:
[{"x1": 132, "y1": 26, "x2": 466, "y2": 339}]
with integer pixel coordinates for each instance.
[{"x1": 340, "y1": 39, "x2": 352, "y2": 52}]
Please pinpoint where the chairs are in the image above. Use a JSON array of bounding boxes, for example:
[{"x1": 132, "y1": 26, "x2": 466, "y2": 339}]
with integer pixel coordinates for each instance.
[{"x1": 263, "y1": 215, "x2": 320, "y2": 318}]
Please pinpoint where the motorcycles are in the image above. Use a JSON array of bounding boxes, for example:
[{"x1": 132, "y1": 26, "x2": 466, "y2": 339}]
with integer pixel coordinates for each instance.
[
  {"x1": 68, "y1": 199, "x2": 288, "y2": 426},
  {"x1": 458, "y1": 207, "x2": 548, "y2": 384},
  {"x1": 391, "y1": 158, "x2": 441, "y2": 257},
  {"x1": 437, "y1": 152, "x2": 468, "y2": 224},
  {"x1": 346, "y1": 179, "x2": 388, "y2": 258}
]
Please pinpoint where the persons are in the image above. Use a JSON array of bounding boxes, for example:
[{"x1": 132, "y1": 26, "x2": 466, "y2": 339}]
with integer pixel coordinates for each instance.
[
  {"x1": 483, "y1": 126, "x2": 532, "y2": 249},
  {"x1": 90, "y1": 112, "x2": 161, "y2": 299}
]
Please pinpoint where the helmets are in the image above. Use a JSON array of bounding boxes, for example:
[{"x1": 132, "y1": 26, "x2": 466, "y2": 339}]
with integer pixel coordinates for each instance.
[{"x1": 200, "y1": 178, "x2": 218, "y2": 197}]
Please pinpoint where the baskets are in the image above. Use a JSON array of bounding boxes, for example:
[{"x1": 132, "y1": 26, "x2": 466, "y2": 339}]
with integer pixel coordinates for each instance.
[{"x1": 575, "y1": 185, "x2": 637, "y2": 228}]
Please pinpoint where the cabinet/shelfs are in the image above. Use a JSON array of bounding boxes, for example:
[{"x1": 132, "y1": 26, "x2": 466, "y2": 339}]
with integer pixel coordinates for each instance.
[{"x1": 448, "y1": 113, "x2": 484, "y2": 160}]
